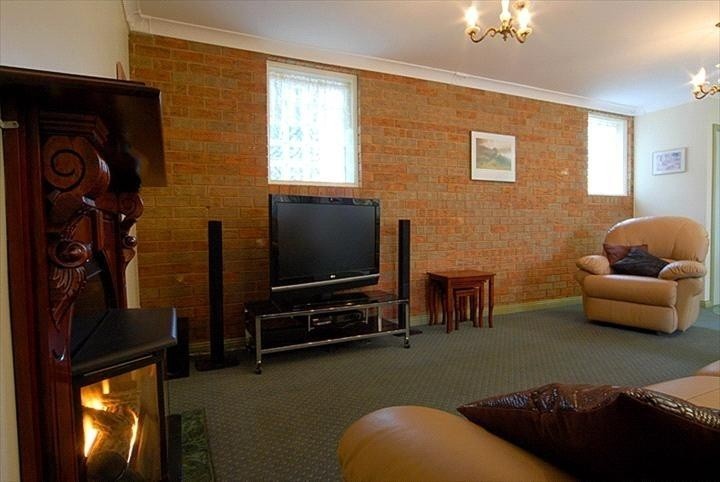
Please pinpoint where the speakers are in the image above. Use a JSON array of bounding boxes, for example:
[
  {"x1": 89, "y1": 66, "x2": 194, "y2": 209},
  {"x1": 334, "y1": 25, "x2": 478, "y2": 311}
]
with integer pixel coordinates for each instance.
[
  {"x1": 392, "y1": 220, "x2": 423, "y2": 336},
  {"x1": 195, "y1": 220, "x2": 240, "y2": 373},
  {"x1": 167, "y1": 317, "x2": 189, "y2": 380}
]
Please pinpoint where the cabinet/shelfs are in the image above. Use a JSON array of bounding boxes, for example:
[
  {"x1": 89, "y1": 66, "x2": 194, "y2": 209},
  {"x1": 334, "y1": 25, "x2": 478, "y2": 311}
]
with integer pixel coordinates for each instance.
[{"x1": 244, "y1": 290, "x2": 410, "y2": 374}]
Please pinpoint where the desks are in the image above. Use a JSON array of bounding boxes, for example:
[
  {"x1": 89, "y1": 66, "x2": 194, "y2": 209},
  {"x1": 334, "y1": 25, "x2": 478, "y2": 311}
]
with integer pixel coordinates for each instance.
[{"x1": 426, "y1": 270, "x2": 496, "y2": 334}]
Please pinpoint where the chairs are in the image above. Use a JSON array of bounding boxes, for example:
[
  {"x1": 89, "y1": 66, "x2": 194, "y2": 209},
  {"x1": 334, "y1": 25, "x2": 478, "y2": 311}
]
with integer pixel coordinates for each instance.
[{"x1": 573, "y1": 215, "x2": 709, "y2": 336}]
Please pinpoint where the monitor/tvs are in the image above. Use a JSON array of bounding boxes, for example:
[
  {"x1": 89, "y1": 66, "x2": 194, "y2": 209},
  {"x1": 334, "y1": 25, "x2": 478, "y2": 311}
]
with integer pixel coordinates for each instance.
[{"x1": 269, "y1": 194, "x2": 380, "y2": 309}]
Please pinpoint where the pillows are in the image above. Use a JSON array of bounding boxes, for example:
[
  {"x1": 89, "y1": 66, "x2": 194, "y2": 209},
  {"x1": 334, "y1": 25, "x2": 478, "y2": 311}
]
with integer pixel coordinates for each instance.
[
  {"x1": 610, "y1": 247, "x2": 670, "y2": 278},
  {"x1": 603, "y1": 244, "x2": 648, "y2": 273},
  {"x1": 457, "y1": 383, "x2": 720, "y2": 482}
]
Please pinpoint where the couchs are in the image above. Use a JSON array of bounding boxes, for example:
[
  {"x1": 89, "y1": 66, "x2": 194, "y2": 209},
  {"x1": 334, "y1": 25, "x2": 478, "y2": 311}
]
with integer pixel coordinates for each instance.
[{"x1": 336, "y1": 360, "x2": 720, "y2": 481}]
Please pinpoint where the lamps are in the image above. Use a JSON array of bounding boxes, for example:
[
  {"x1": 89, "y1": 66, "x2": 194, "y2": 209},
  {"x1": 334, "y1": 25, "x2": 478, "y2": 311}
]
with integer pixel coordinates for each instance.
[
  {"x1": 456, "y1": 0, "x2": 535, "y2": 44},
  {"x1": 688, "y1": 21, "x2": 720, "y2": 99}
]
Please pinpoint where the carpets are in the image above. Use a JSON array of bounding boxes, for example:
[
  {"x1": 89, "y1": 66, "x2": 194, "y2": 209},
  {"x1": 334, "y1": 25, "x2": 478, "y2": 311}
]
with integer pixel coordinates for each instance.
[{"x1": 168, "y1": 407, "x2": 215, "y2": 482}]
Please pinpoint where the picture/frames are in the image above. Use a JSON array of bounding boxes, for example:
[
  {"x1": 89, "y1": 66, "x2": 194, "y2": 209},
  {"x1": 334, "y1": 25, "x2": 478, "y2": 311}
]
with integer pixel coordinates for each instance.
[
  {"x1": 469, "y1": 130, "x2": 517, "y2": 183},
  {"x1": 652, "y1": 148, "x2": 687, "y2": 176}
]
